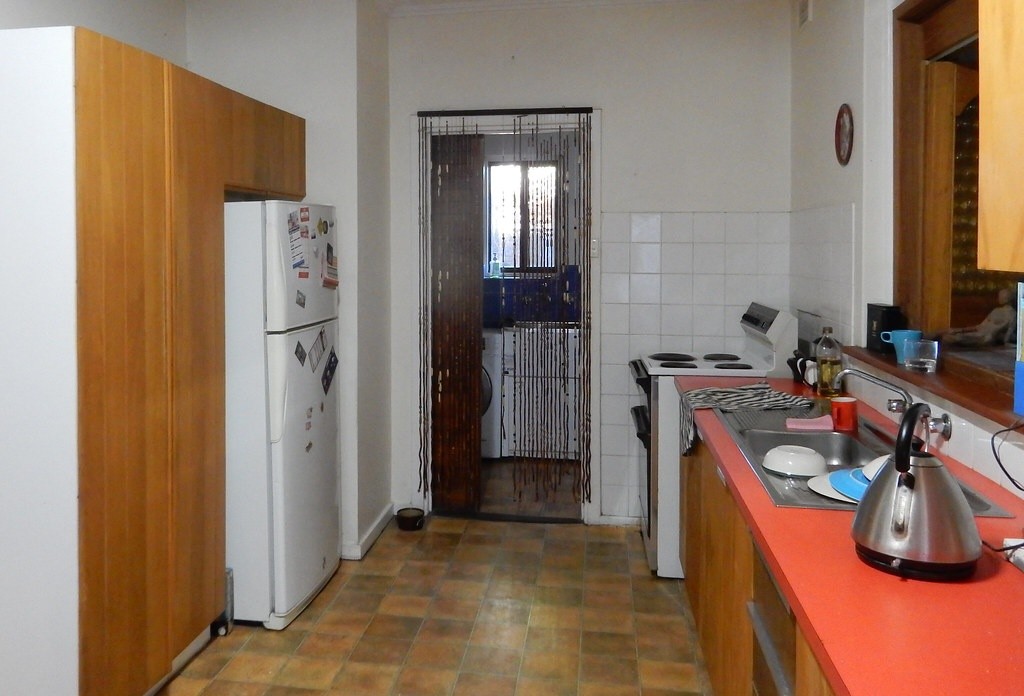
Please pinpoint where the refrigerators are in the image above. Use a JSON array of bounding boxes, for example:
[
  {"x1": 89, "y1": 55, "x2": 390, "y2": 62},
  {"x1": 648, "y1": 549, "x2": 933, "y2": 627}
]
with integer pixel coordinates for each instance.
[{"x1": 226, "y1": 199, "x2": 341, "y2": 630}]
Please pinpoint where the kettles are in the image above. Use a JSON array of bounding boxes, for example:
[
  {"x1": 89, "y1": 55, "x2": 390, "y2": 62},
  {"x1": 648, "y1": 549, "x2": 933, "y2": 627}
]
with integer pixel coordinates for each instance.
[{"x1": 851, "y1": 402, "x2": 982, "y2": 582}]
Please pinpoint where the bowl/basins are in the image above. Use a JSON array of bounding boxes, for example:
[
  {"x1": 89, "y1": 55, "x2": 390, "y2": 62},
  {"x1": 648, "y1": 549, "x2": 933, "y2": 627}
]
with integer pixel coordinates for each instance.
[
  {"x1": 762, "y1": 444, "x2": 829, "y2": 477},
  {"x1": 862, "y1": 454, "x2": 891, "y2": 480}
]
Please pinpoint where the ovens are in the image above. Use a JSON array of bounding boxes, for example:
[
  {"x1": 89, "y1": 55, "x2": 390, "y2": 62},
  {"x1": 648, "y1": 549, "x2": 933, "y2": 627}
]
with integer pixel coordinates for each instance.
[{"x1": 626, "y1": 359, "x2": 658, "y2": 574}]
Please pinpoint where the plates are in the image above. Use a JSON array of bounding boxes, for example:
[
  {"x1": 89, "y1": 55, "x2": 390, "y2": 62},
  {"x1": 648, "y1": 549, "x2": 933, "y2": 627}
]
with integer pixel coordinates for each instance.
[{"x1": 807, "y1": 468, "x2": 870, "y2": 503}]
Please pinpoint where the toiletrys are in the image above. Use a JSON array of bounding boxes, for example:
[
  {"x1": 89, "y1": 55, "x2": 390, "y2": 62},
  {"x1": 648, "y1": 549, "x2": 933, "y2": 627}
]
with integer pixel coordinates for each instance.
[{"x1": 489, "y1": 253, "x2": 500, "y2": 276}]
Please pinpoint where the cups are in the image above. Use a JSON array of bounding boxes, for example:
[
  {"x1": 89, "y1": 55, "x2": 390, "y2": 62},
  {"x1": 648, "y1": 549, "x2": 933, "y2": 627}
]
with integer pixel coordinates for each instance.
[
  {"x1": 880, "y1": 330, "x2": 923, "y2": 363},
  {"x1": 904, "y1": 339, "x2": 938, "y2": 372},
  {"x1": 797, "y1": 358, "x2": 818, "y2": 386},
  {"x1": 831, "y1": 396, "x2": 858, "y2": 432}
]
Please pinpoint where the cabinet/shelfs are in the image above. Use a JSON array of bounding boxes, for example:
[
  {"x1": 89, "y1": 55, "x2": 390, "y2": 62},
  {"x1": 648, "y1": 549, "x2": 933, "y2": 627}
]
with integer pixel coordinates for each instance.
[
  {"x1": 0, "y1": 24, "x2": 306, "y2": 696},
  {"x1": 502, "y1": 328, "x2": 583, "y2": 459},
  {"x1": 679, "y1": 421, "x2": 835, "y2": 696}
]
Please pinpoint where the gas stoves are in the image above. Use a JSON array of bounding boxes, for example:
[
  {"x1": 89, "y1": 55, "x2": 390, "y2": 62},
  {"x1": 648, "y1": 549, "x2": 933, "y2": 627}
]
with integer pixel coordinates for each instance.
[{"x1": 641, "y1": 302, "x2": 800, "y2": 378}]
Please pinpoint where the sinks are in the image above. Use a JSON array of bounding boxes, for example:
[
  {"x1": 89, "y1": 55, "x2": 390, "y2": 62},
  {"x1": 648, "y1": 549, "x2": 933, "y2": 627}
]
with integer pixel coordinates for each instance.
[{"x1": 738, "y1": 428, "x2": 882, "y2": 469}]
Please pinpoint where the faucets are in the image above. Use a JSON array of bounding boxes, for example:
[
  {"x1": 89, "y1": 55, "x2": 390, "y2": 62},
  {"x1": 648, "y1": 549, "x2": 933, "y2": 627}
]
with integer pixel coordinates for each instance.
[{"x1": 832, "y1": 368, "x2": 914, "y2": 404}]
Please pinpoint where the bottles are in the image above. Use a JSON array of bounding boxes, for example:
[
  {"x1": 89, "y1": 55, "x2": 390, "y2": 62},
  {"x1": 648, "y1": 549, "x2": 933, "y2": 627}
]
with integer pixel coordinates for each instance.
[{"x1": 815, "y1": 326, "x2": 843, "y2": 397}]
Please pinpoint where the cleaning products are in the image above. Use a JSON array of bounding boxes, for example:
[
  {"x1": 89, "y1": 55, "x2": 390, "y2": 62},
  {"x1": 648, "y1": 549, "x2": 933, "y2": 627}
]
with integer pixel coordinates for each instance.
[{"x1": 816, "y1": 327, "x2": 842, "y2": 398}]
